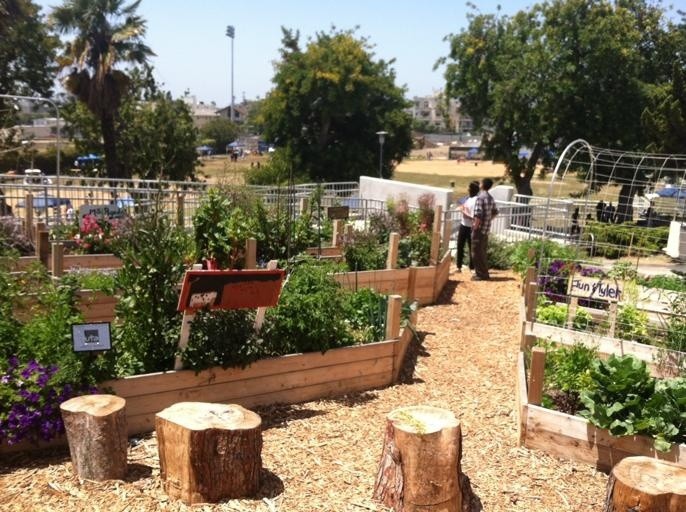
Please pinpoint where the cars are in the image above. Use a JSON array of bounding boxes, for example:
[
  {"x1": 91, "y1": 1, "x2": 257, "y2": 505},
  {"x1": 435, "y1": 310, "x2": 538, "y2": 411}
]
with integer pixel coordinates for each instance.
[{"x1": 23, "y1": 169, "x2": 52, "y2": 191}]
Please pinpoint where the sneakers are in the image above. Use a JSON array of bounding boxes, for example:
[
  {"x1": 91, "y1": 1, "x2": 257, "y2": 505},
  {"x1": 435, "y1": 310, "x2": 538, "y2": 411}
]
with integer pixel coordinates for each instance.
[{"x1": 454, "y1": 268, "x2": 489, "y2": 281}]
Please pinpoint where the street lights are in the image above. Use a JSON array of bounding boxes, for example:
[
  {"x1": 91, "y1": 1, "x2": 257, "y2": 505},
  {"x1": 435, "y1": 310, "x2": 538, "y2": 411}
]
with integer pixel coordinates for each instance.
[
  {"x1": 376, "y1": 130, "x2": 388, "y2": 178},
  {"x1": 226, "y1": 25, "x2": 235, "y2": 121}
]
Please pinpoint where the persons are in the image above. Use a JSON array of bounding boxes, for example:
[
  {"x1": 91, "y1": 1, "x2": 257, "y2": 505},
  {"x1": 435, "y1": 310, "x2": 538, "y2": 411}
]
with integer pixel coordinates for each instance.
[
  {"x1": 451, "y1": 180, "x2": 482, "y2": 274},
  {"x1": 470, "y1": 177, "x2": 500, "y2": 282},
  {"x1": 569, "y1": 198, "x2": 616, "y2": 236}
]
